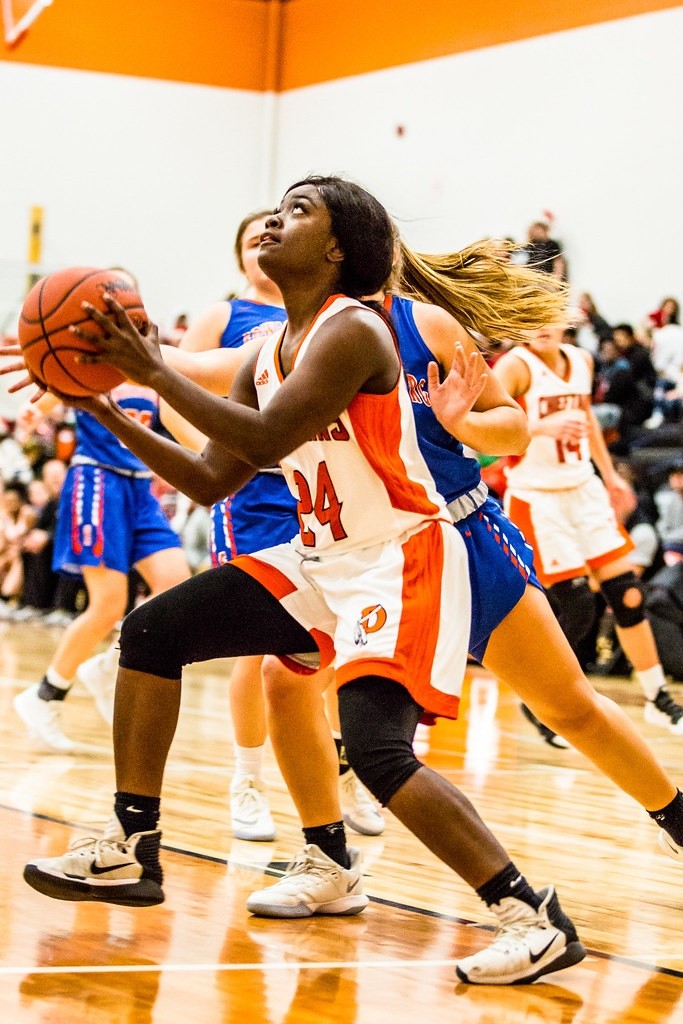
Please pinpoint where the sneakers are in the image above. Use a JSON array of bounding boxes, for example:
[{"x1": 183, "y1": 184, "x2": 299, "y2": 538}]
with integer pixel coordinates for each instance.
[
  {"x1": 644, "y1": 687, "x2": 682, "y2": 733},
  {"x1": 23, "y1": 818, "x2": 164, "y2": 907},
  {"x1": 519, "y1": 702, "x2": 577, "y2": 749},
  {"x1": 657, "y1": 824, "x2": 682, "y2": 860},
  {"x1": 75, "y1": 653, "x2": 118, "y2": 730},
  {"x1": 246, "y1": 843, "x2": 367, "y2": 918},
  {"x1": 228, "y1": 770, "x2": 276, "y2": 840},
  {"x1": 330, "y1": 730, "x2": 385, "y2": 835},
  {"x1": 455, "y1": 883, "x2": 586, "y2": 984},
  {"x1": 11, "y1": 683, "x2": 72, "y2": 752}
]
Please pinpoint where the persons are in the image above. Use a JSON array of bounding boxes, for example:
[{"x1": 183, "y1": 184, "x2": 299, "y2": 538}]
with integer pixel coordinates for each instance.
[
  {"x1": 0, "y1": 387, "x2": 150, "y2": 628},
  {"x1": 10, "y1": 264, "x2": 192, "y2": 753},
  {"x1": 155, "y1": 206, "x2": 389, "y2": 839},
  {"x1": 0, "y1": 177, "x2": 580, "y2": 990},
  {"x1": 490, "y1": 292, "x2": 681, "y2": 750},
  {"x1": 548, "y1": 292, "x2": 683, "y2": 677},
  {"x1": 515, "y1": 222, "x2": 562, "y2": 283},
  {"x1": 3, "y1": 211, "x2": 683, "y2": 916}
]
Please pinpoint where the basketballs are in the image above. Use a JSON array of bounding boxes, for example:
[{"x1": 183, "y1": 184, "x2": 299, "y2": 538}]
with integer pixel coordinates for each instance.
[{"x1": 18, "y1": 265, "x2": 149, "y2": 399}]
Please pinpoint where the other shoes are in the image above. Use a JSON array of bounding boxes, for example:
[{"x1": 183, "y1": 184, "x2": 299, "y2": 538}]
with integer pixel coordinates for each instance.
[{"x1": 0, "y1": 594, "x2": 131, "y2": 637}]
